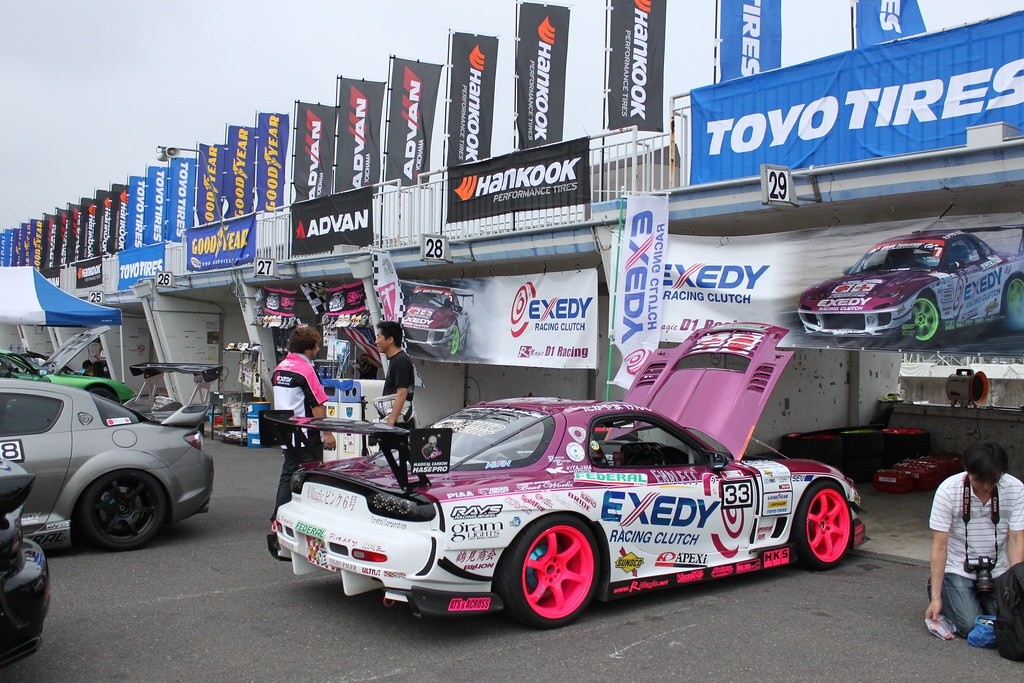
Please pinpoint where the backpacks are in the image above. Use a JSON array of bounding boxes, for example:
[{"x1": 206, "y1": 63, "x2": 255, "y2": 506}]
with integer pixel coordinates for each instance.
[{"x1": 994, "y1": 562, "x2": 1024, "y2": 662}]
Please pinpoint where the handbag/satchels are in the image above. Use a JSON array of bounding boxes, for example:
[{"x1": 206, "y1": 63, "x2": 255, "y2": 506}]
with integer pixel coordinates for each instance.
[
  {"x1": 966, "y1": 615, "x2": 996, "y2": 649},
  {"x1": 372, "y1": 393, "x2": 412, "y2": 424}
]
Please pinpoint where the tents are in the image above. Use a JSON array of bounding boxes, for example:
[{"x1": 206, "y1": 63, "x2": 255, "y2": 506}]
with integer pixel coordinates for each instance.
[{"x1": 0, "y1": 266, "x2": 124, "y2": 384}]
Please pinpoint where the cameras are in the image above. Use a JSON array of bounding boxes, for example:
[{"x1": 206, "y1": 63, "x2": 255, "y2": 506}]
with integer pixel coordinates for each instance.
[{"x1": 964, "y1": 556, "x2": 996, "y2": 592}]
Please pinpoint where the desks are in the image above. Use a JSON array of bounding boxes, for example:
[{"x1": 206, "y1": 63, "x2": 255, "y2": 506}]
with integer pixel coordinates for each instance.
[{"x1": 210, "y1": 391, "x2": 253, "y2": 440}]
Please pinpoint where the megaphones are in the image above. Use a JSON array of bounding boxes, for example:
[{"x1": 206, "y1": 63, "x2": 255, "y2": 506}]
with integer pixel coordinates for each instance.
[
  {"x1": 162, "y1": 147, "x2": 180, "y2": 158},
  {"x1": 156, "y1": 151, "x2": 169, "y2": 162}
]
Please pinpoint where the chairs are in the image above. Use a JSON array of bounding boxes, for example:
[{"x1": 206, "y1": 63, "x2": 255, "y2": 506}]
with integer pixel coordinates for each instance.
[{"x1": 950, "y1": 246, "x2": 968, "y2": 264}]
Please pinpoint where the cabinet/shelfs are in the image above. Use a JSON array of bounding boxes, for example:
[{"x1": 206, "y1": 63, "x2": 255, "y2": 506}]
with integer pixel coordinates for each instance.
[{"x1": 311, "y1": 346, "x2": 357, "y2": 379}]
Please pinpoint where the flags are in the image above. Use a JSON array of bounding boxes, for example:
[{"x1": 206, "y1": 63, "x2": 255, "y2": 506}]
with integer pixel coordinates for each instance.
[
  {"x1": 519, "y1": 1, "x2": 927, "y2": 147},
  {"x1": 257, "y1": 31, "x2": 499, "y2": 211},
  {"x1": 125, "y1": 126, "x2": 256, "y2": 250},
  {"x1": 0, "y1": 183, "x2": 129, "y2": 269}
]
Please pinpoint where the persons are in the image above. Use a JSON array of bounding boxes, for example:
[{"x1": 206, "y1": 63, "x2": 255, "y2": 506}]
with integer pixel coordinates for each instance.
[
  {"x1": 926, "y1": 440, "x2": 1024, "y2": 640},
  {"x1": 375, "y1": 321, "x2": 415, "y2": 452},
  {"x1": 81, "y1": 360, "x2": 107, "y2": 378},
  {"x1": 270, "y1": 326, "x2": 336, "y2": 532}
]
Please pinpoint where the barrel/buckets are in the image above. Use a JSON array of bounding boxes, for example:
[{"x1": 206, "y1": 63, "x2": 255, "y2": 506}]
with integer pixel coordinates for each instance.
[{"x1": 246, "y1": 401, "x2": 271, "y2": 447}]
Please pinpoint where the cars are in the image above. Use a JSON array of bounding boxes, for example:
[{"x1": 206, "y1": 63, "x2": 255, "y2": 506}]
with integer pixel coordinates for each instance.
[
  {"x1": 405, "y1": 288, "x2": 469, "y2": 357},
  {"x1": 267, "y1": 322, "x2": 869, "y2": 631},
  {"x1": 0, "y1": 349, "x2": 137, "y2": 407},
  {"x1": 799, "y1": 223, "x2": 1024, "y2": 351},
  {"x1": 0, "y1": 377, "x2": 214, "y2": 553}
]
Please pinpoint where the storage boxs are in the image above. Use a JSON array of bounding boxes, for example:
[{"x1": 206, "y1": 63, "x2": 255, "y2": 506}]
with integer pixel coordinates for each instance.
[{"x1": 872, "y1": 455, "x2": 961, "y2": 493}]
